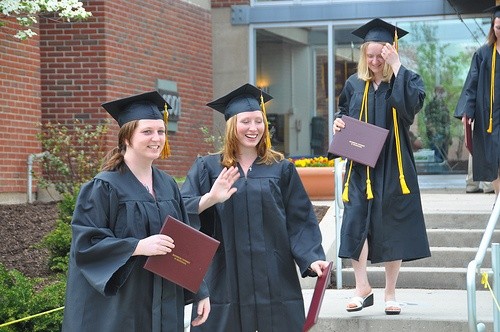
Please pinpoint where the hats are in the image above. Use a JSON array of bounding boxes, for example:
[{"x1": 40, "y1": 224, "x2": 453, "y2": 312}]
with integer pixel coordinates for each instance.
[
  {"x1": 207, "y1": 82, "x2": 275, "y2": 150},
  {"x1": 482, "y1": 4, "x2": 500, "y2": 18},
  {"x1": 100, "y1": 89, "x2": 173, "y2": 160},
  {"x1": 352, "y1": 18, "x2": 409, "y2": 53}
]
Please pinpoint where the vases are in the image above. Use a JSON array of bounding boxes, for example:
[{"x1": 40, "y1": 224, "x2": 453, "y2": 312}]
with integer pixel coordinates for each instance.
[{"x1": 294, "y1": 167, "x2": 346, "y2": 200}]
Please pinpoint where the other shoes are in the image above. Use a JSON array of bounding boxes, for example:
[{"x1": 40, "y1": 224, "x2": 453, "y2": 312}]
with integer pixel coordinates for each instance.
[
  {"x1": 484, "y1": 189, "x2": 494, "y2": 193},
  {"x1": 465, "y1": 187, "x2": 483, "y2": 192}
]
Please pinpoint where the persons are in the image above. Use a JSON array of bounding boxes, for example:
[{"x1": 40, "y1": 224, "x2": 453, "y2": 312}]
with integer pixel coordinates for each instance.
[
  {"x1": 180, "y1": 83, "x2": 331, "y2": 332},
  {"x1": 62, "y1": 92, "x2": 210, "y2": 332},
  {"x1": 425, "y1": 86, "x2": 452, "y2": 162},
  {"x1": 453, "y1": 5, "x2": 500, "y2": 198},
  {"x1": 327, "y1": 18, "x2": 431, "y2": 315},
  {"x1": 466, "y1": 153, "x2": 495, "y2": 193}
]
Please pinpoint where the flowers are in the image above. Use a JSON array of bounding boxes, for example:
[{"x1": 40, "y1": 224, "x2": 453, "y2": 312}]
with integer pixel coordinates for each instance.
[{"x1": 288, "y1": 156, "x2": 343, "y2": 167}]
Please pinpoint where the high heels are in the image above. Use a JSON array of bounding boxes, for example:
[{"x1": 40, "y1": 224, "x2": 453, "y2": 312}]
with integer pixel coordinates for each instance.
[
  {"x1": 345, "y1": 291, "x2": 374, "y2": 311},
  {"x1": 384, "y1": 293, "x2": 401, "y2": 314}
]
